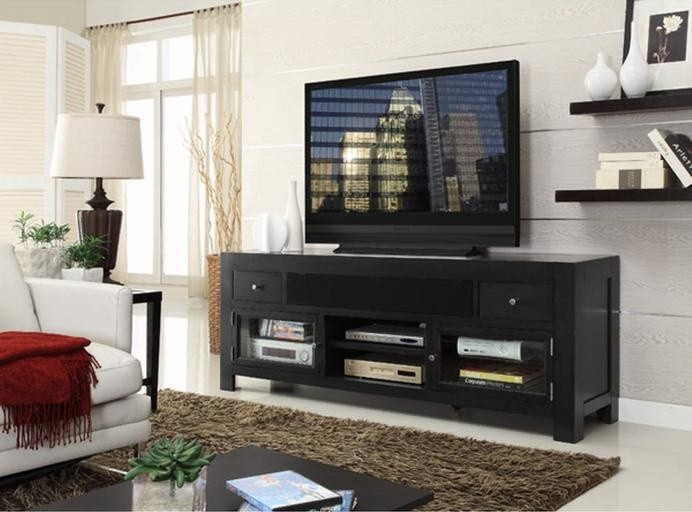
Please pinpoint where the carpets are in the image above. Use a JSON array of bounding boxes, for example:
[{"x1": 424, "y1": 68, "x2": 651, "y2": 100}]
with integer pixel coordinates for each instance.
[{"x1": 1, "y1": 386, "x2": 620, "y2": 510}]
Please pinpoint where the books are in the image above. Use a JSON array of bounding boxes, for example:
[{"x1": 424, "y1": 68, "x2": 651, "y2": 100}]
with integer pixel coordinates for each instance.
[
  {"x1": 597, "y1": 127, "x2": 691, "y2": 192},
  {"x1": 225, "y1": 469, "x2": 343, "y2": 512},
  {"x1": 457, "y1": 362, "x2": 544, "y2": 391},
  {"x1": 239, "y1": 489, "x2": 358, "y2": 512}
]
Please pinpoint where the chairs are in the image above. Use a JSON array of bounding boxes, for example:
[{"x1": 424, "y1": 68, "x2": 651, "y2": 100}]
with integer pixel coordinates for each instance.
[{"x1": 0, "y1": 245, "x2": 153, "y2": 477}]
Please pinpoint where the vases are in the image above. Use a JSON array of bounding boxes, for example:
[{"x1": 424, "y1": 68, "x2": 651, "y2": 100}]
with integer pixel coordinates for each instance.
[
  {"x1": 583, "y1": 52, "x2": 617, "y2": 102},
  {"x1": 263, "y1": 172, "x2": 306, "y2": 256},
  {"x1": 618, "y1": 17, "x2": 652, "y2": 99}
]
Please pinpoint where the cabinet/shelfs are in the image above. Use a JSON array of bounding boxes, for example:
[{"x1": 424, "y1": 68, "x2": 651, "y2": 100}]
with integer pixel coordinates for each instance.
[
  {"x1": 219, "y1": 250, "x2": 621, "y2": 442},
  {"x1": 554, "y1": 88, "x2": 692, "y2": 204}
]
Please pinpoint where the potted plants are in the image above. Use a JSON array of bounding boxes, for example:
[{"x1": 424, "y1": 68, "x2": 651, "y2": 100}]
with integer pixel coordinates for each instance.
[
  {"x1": 9, "y1": 210, "x2": 72, "y2": 281},
  {"x1": 60, "y1": 233, "x2": 111, "y2": 282},
  {"x1": 124, "y1": 434, "x2": 218, "y2": 511}
]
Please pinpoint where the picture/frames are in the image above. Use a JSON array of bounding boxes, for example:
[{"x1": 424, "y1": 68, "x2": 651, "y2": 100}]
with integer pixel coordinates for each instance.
[{"x1": 616, "y1": 0, "x2": 692, "y2": 94}]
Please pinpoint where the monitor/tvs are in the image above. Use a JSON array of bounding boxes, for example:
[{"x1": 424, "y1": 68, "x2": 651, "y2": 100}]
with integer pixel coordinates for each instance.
[{"x1": 306, "y1": 60, "x2": 519, "y2": 255}]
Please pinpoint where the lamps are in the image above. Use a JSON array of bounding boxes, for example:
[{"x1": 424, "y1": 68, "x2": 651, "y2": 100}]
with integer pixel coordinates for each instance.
[{"x1": 48, "y1": 101, "x2": 146, "y2": 290}]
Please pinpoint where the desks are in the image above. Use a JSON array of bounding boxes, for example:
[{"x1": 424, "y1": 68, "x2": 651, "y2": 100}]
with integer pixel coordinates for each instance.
[{"x1": 127, "y1": 286, "x2": 162, "y2": 416}]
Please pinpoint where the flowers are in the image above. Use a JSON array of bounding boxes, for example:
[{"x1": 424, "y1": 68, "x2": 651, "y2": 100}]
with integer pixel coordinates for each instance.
[{"x1": 651, "y1": 14, "x2": 684, "y2": 63}]
